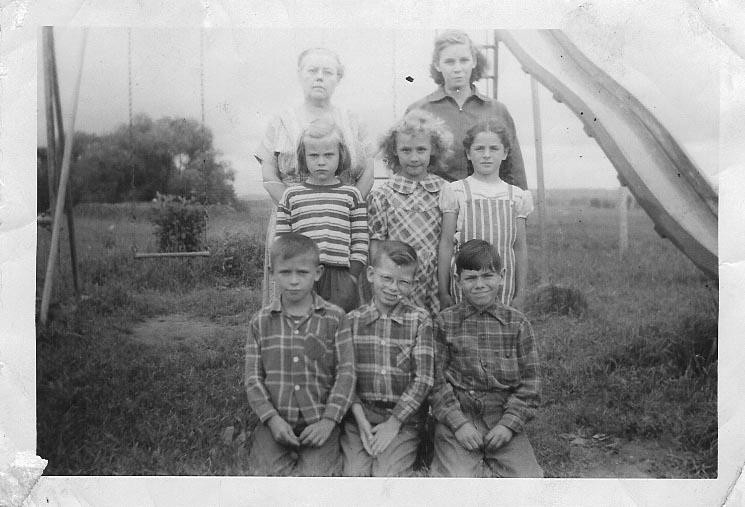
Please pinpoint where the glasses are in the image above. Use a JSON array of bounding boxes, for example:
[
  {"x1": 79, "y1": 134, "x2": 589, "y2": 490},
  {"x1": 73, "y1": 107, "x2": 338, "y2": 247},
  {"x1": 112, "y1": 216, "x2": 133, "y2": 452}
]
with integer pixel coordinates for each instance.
[{"x1": 373, "y1": 268, "x2": 414, "y2": 290}]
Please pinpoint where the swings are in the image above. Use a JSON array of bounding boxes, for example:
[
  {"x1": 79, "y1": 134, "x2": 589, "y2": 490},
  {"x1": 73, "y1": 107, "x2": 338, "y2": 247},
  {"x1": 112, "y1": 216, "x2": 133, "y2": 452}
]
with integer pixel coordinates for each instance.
[{"x1": 129, "y1": 25, "x2": 213, "y2": 258}]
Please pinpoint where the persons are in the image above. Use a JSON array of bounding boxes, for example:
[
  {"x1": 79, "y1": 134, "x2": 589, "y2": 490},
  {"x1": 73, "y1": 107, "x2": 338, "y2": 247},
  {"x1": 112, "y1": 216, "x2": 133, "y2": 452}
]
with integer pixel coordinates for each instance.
[
  {"x1": 428, "y1": 238, "x2": 544, "y2": 478},
  {"x1": 436, "y1": 117, "x2": 534, "y2": 313},
  {"x1": 256, "y1": 46, "x2": 377, "y2": 205},
  {"x1": 401, "y1": 26, "x2": 527, "y2": 191},
  {"x1": 340, "y1": 239, "x2": 434, "y2": 477},
  {"x1": 239, "y1": 232, "x2": 358, "y2": 477},
  {"x1": 273, "y1": 118, "x2": 371, "y2": 311},
  {"x1": 365, "y1": 109, "x2": 451, "y2": 312}
]
